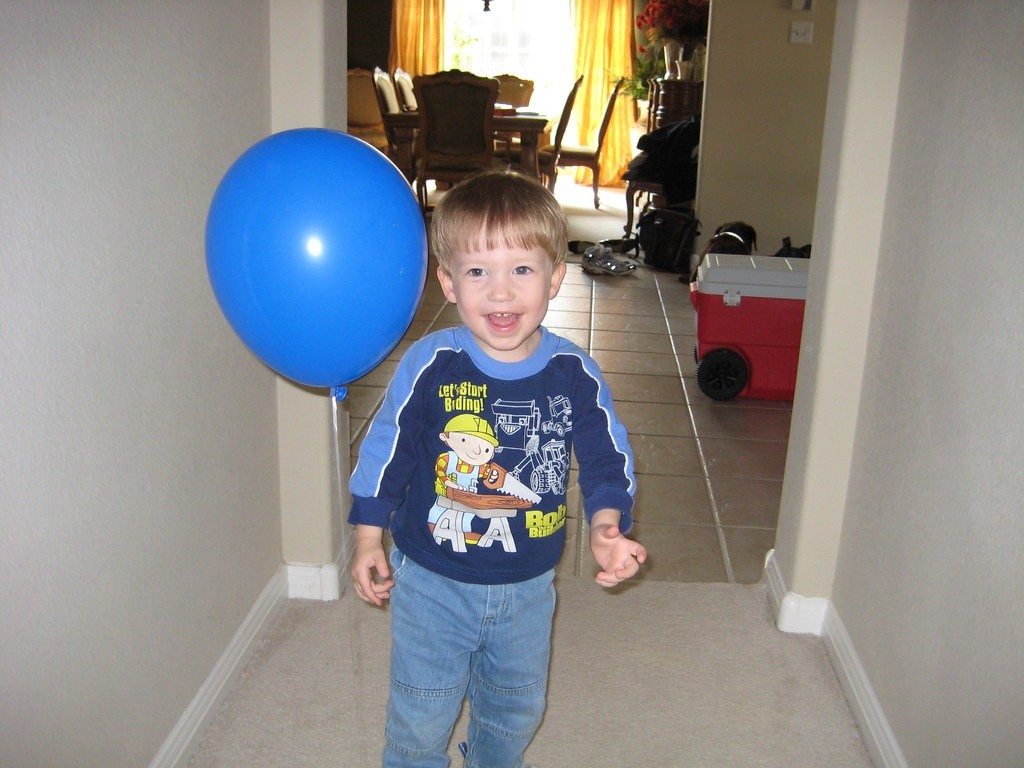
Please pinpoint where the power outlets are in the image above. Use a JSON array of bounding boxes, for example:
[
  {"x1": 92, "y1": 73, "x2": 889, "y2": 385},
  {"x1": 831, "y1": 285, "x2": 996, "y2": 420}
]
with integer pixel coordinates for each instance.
[{"x1": 789, "y1": 22, "x2": 814, "y2": 44}]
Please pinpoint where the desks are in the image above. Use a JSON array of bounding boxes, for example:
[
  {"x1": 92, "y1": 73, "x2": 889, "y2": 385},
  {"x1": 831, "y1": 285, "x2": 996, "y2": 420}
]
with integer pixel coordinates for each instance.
[{"x1": 379, "y1": 109, "x2": 549, "y2": 187}]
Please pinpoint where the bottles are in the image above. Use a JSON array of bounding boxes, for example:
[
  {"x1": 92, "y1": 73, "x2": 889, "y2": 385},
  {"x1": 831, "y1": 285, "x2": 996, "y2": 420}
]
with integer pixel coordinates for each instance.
[{"x1": 675, "y1": 60, "x2": 696, "y2": 81}]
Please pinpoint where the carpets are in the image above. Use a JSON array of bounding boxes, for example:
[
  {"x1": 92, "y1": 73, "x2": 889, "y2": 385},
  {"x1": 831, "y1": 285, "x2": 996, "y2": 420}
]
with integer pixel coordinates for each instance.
[{"x1": 184, "y1": 563, "x2": 875, "y2": 768}]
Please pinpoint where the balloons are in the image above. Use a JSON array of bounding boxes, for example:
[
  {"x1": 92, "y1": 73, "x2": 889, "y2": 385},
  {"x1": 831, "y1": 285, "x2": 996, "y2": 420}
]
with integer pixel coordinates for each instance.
[{"x1": 206, "y1": 128, "x2": 428, "y2": 398}]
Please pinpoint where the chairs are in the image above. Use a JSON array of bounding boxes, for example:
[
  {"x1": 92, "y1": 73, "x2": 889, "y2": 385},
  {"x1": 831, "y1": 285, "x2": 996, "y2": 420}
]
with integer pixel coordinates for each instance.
[
  {"x1": 538, "y1": 76, "x2": 627, "y2": 210},
  {"x1": 622, "y1": 179, "x2": 666, "y2": 238},
  {"x1": 345, "y1": 67, "x2": 585, "y2": 218}
]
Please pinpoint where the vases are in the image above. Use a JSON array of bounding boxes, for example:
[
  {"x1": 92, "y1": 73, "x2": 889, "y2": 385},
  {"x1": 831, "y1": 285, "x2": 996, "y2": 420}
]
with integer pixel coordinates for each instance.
[
  {"x1": 675, "y1": 60, "x2": 697, "y2": 80},
  {"x1": 664, "y1": 41, "x2": 684, "y2": 79}
]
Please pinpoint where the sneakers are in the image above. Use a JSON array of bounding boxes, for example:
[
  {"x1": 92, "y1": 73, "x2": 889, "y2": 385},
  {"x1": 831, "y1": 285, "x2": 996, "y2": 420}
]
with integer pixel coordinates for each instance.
[{"x1": 584, "y1": 248, "x2": 636, "y2": 276}]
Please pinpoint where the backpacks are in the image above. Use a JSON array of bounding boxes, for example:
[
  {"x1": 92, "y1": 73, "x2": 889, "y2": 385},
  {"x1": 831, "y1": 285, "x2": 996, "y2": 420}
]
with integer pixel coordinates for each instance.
[
  {"x1": 688, "y1": 221, "x2": 757, "y2": 283},
  {"x1": 641, "y1": 207, "x2": 698, "y2": 272}
]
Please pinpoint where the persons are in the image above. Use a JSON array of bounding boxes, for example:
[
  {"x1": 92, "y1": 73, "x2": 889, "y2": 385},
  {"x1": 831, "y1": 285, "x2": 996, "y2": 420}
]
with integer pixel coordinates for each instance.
[{"x1": 348, "y1": 173, "x2": 647, "y2": 768}]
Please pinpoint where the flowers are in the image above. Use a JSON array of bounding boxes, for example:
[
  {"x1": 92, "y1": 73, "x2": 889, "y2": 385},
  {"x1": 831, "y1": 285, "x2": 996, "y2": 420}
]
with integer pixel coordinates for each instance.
[{"x1": 637, "y1": 0, "x2": 710, "y2": 61}]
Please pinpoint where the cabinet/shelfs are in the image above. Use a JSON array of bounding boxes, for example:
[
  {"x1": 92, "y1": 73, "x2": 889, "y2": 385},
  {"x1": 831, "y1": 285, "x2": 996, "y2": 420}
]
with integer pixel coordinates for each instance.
[{"x1": 645, "y1": 78, "x2": 704, "y2": 137}]
